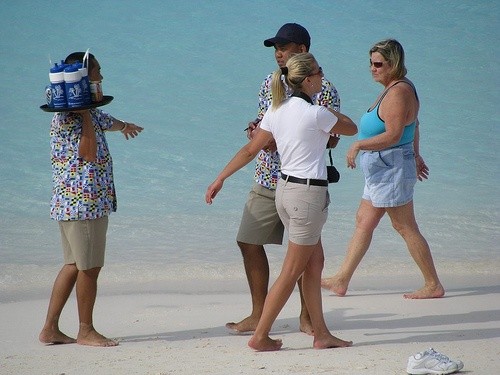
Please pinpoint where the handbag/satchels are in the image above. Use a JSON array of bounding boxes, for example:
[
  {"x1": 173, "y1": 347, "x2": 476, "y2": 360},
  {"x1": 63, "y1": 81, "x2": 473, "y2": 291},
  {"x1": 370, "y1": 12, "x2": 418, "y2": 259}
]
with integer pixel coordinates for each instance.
[{"x1": 291, "y1": 92, "x2": 340, "y2": 183}]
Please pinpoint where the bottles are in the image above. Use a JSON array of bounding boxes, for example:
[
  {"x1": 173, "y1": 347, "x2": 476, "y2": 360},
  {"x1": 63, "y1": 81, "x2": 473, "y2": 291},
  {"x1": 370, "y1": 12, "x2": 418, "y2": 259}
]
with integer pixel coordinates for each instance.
[
  {"x1": 49, "y1": 63, "x2": 67, "y2": 108},
  {"x1": 72, "y1": 60, "x2": 91, "y2": 106},
  {"x1": 59, "y1": 60, "x2": 71, "y2": 71},
  {"x1": 63, "y1": 64, "x2": 83, "y2": 108}
]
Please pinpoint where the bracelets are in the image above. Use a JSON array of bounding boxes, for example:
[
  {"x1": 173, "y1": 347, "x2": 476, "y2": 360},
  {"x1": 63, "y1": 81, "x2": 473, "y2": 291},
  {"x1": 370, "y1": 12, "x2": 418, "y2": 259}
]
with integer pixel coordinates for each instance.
[{"x1": 120, "y1": 119, "x2": 128, "y2": 131}]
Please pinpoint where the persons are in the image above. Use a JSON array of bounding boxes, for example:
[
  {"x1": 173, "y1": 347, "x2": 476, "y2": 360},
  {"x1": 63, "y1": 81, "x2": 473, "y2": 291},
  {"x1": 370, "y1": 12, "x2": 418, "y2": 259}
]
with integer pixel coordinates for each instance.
[
  {"x1": 38, "y1": 50, "x2": 144, "y2": 348},
  {"x1": 224, "y1": 23, "x2": 342, "y2": 336},
  {"x1": 205, "y1": 51, "x2": 359, "y2": 352},
  {"x1": 321, "y1": 38, "x2": 446, "y2": 300}
]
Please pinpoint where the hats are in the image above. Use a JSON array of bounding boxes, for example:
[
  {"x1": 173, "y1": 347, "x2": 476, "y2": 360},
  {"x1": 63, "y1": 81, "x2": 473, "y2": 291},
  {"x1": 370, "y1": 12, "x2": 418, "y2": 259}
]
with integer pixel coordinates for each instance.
[{"x1": 264, "y1": 22, "x2": 310, "y2": 50}]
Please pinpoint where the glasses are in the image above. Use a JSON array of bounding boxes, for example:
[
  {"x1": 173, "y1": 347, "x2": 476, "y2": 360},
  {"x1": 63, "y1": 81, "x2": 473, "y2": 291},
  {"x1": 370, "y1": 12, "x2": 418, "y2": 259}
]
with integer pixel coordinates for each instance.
[{"x1": 370, "y1": 59, "x2": 390, "y2": 67}]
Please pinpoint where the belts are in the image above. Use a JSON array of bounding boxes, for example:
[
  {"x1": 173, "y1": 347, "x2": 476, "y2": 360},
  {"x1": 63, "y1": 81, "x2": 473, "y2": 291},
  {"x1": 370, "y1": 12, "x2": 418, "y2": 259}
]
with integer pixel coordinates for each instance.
[{"x1": 280, "y1": 172, "x2": 328, "y2": 187}]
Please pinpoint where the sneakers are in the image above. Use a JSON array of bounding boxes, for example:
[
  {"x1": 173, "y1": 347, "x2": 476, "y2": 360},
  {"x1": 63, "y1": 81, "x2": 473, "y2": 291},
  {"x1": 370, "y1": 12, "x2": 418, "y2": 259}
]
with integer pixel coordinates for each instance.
[{"x1": 407, "y1": 348, "x2": 464, "y2": 375}]
